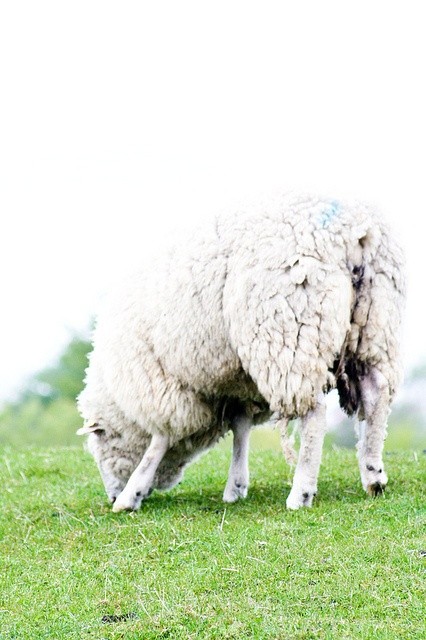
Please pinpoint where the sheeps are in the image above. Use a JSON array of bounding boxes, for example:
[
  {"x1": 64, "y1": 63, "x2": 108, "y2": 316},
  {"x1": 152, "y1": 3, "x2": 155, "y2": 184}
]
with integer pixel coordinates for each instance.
[{"x1": 74, "y1": 194, "x2": 411, "y2": 516}]
